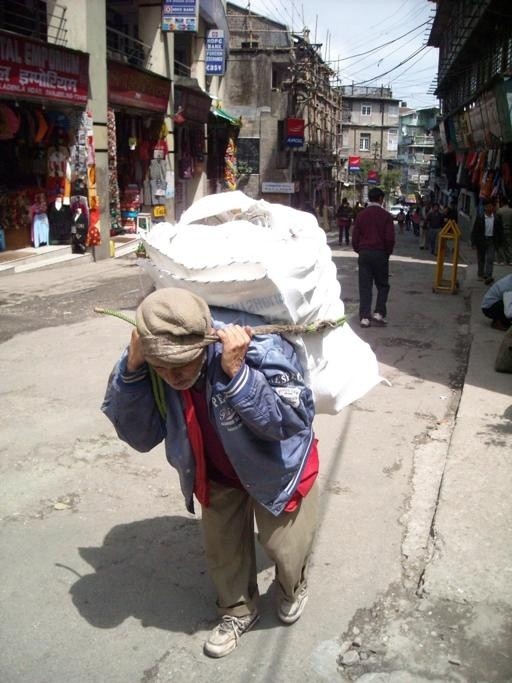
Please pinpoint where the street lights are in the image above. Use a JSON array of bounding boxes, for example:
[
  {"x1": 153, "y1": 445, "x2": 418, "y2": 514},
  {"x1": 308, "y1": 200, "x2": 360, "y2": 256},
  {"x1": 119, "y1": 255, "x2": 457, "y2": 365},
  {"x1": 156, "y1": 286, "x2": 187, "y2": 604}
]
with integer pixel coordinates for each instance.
[{"x1": 335, "y1": 145, "x2": 352, "y2": 220}]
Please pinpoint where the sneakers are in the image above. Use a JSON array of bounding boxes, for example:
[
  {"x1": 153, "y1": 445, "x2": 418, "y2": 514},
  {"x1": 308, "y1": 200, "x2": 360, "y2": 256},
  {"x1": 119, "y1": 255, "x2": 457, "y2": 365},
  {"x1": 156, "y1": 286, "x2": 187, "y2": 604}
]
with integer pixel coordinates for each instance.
[
  {"x1": 277, "y1": 580, "x2": 308, "y2": 624},
  {"x1": 360, "y1": 318, "x2": 371, "y2": 328},
  {"x1": 204, "y1": 608, "x2": 260, "y2": 658},
  {"x1": 372, "y1": 313, "x2": 388, "y2": 324}
]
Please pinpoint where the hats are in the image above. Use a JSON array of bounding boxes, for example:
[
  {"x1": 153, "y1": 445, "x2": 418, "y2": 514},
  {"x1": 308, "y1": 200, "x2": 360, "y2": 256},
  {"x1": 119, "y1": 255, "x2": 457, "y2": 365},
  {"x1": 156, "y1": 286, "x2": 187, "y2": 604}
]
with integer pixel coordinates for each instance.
[{"x1": 136, "y1": 288, "x2": 212, "y2": 369}]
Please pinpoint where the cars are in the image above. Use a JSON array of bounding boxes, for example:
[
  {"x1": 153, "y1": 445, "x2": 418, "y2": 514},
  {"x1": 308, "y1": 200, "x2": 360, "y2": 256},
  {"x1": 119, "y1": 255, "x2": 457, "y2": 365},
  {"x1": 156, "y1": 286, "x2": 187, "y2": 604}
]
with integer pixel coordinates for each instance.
[{"x1": 389, "y1": 205, "x2": 406, "y2": 218}]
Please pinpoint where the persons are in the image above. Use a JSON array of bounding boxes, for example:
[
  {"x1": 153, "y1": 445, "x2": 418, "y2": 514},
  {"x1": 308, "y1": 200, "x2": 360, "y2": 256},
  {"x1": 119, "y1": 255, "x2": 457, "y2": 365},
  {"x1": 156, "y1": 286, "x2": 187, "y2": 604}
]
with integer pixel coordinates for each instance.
[
  {"x1": 470, "y1": 199, "x2": 504, "y2": 286},
  {"x1": 99, "y1": 287, "x2": 323, "y2": 658},
  {"x1": 351, "y1": 187, "x2": 395, "y2": 328},
  {"x1": 493, "y1": 196, "x2": 512, "y2": 267},
  {"x1": 481, "y1": 273, "x2": 512, "y2": 331},
  {"x1": 302, "y1": 198, "x2": 464, "y2": 256}
]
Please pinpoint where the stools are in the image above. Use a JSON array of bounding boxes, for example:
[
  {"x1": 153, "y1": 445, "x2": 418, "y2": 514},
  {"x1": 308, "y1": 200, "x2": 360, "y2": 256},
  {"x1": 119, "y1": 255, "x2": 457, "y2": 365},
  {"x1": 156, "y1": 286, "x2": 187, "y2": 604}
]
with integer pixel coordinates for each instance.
[{"x1": 137, "y1": 213, "x2": 151, "y2": 234}]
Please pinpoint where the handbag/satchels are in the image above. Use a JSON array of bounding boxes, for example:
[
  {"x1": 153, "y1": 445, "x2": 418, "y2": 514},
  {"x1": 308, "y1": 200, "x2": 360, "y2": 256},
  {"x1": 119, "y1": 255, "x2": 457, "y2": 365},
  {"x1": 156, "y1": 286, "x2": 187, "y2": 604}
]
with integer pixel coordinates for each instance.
[{"x1": 207, "y1": 477, "x2": 250, "y2": 520}]
